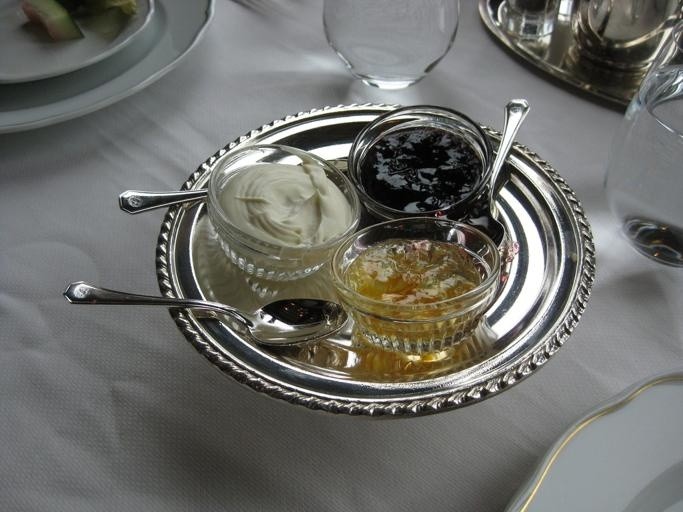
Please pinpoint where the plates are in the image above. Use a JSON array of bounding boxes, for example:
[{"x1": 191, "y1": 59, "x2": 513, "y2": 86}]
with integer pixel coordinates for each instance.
[
  {"x1": 3, "y1": 0, "x2": 214, "y2": 136},
  {"x1": 477, "y1": 0, "x2": 628, "y2": 108},
  {"x1": 154, "y1": 100, "x2": 596, "y2": 419},
  {"x1": 494, "y1": 365, "x2": 683, "y2": 512}
]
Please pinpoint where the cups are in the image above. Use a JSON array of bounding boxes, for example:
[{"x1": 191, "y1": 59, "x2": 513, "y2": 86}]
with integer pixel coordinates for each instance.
[
  {"x1": 497, "y1": 1, "x2": 683, "y2": 73},
  {"x1": 608, "y1": 22, "x2": 682, "y2": 268},
  {"x1": 322, "y1": 1, "x2": 460, "y2": 92}
]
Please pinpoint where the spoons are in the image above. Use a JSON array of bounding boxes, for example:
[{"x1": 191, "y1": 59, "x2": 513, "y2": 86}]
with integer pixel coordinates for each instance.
[
  {"x1": 448, "y1": 97, "x2": 533, "y2": 254},
  {"x1": 65, "y1": 281, "x2": 351, "y2": 348}
]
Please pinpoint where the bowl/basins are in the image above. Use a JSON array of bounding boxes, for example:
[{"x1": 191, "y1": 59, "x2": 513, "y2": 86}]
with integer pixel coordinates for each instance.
[
  {"x1": 337, "y1": 217, "x2": 501, "y2": 357},
  {"x1": 345, "y1": 101, "x2": 493, "y2": 221},
  {"x1": 204, "y1": 142, "x2": 360, "y2": 283}
]
